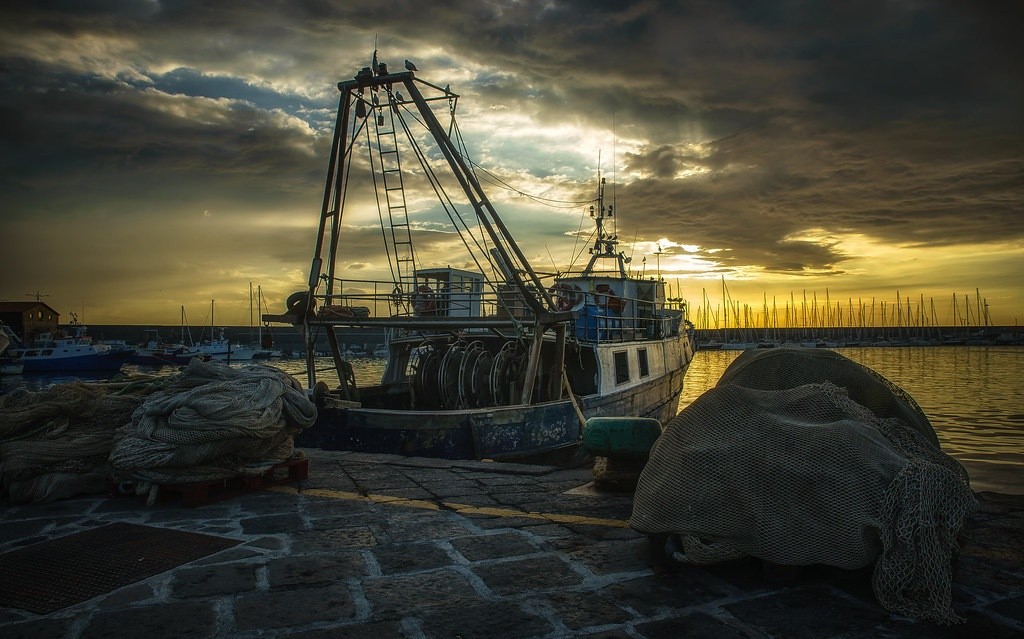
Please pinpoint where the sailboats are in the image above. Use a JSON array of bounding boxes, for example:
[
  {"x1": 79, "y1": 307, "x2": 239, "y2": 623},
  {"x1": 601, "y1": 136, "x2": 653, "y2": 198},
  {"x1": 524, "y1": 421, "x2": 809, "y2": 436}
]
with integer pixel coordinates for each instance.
[{"x1": 669, "y1": 273, "x2": 1024, "y2": 350}]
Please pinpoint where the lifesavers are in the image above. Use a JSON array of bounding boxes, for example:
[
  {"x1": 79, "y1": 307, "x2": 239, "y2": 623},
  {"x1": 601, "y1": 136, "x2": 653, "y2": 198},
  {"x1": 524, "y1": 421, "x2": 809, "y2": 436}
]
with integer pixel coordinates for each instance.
[
  {"x1": 411, "y1": 285, "x2": 436, "y2": 313},
  {"x1": 287, "y1": 292, "x2": 315, "y2": 311},
  {"x1": 548, "y1": 283, "x2": 575, "y2": 312},
  {"x1": 594, "y1": 287, "x2": 615, "y2": 308},
  {"x1": 570, "y1": 284, "x2": 582, "y2": 304}
]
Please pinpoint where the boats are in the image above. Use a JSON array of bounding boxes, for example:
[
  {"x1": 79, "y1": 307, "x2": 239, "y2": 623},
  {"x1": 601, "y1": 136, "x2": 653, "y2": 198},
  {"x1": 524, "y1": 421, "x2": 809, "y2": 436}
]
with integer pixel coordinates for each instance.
[
  {"x1": 258, "y1": 26, "x2": 700, "y2": 457},
  {"x1": 1, "y1": 280, "x2": 431, "y2": 371}
]
